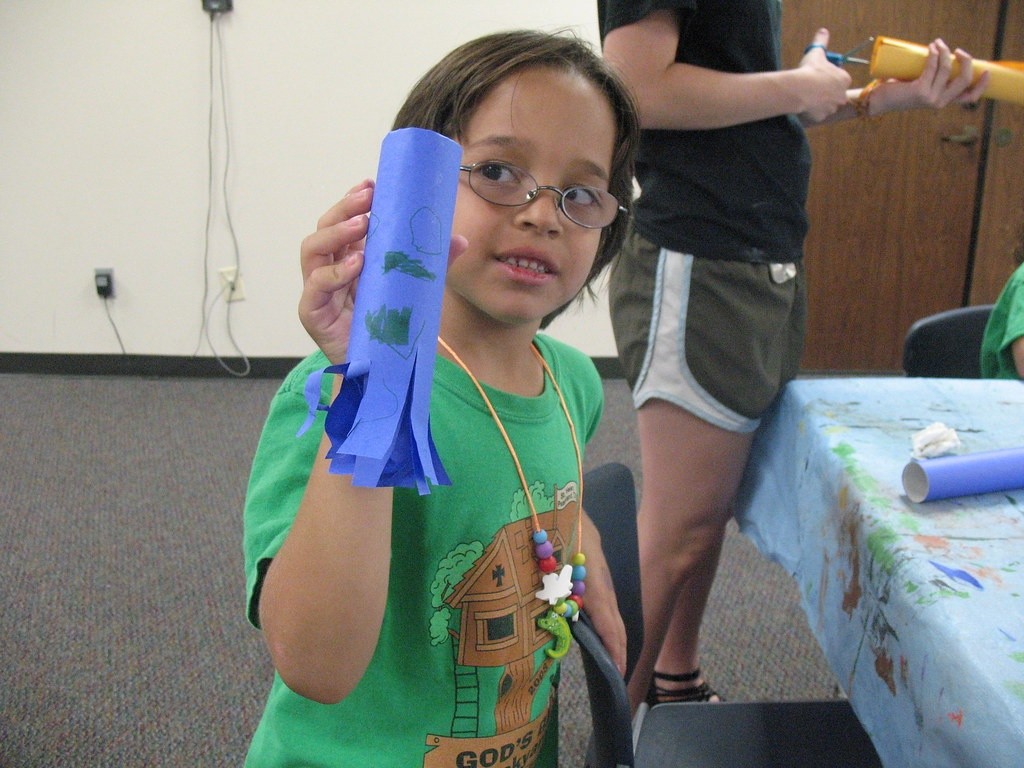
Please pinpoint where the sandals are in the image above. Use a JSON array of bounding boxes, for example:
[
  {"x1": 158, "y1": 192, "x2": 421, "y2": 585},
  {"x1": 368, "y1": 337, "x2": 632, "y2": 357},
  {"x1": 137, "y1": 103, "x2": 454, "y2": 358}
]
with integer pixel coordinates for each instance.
[{"x1": 644, "y1": 667, "x2": 726, "y2": 708}]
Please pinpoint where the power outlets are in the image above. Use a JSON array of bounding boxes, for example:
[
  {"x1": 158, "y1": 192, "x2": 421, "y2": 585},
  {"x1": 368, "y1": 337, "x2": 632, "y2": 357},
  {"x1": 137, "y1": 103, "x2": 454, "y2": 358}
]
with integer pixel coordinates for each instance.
[{"x1": 218, "y1": 267, "x2": 246, "y2": 303}]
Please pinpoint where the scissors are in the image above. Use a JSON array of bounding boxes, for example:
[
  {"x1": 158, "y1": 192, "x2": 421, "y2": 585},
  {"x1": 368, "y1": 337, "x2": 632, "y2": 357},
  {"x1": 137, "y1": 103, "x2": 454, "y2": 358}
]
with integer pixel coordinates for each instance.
[{"x1": 803, "y1": 36, "x2": 875, "y2": 69}]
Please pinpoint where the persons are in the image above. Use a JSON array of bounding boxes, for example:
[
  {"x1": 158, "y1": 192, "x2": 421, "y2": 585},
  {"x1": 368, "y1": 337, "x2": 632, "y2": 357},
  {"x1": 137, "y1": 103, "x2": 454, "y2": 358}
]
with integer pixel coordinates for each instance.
[
  {"x1": 981, "y1": 232, "x2": 1024, "y2": 379},
  {"x1": 243, "y1": 29, "x2": 643, "y2": 768},
  {"x1": 596, "y1": 0, "x2": 989, "y2": 708}
]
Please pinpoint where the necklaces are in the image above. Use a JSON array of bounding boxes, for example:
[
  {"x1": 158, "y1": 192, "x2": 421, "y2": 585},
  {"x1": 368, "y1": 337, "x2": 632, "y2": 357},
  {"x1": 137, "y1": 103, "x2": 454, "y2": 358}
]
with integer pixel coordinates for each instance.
[{"x1": 437, "y1": 334, "x2": 588, "y2": 660}]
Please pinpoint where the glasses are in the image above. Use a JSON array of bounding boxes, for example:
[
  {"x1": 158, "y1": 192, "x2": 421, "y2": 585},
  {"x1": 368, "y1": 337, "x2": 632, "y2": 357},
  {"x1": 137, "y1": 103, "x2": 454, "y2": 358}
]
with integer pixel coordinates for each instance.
[{"x1": 460, "y1": 159, "x2": 629, "y2": 230}]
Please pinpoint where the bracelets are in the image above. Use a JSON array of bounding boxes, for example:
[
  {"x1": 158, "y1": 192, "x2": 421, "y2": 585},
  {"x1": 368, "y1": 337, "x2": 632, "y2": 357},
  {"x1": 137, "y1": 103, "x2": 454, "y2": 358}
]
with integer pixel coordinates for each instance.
[{"x1": 858, "y1": 76, "x2": 879, "y2": 121}]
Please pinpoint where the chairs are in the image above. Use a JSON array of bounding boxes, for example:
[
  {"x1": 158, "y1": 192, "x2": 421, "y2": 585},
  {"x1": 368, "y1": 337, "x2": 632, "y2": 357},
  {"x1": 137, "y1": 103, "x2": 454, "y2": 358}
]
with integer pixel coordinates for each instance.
[
  {"x1": 569, "y1": 463, "x2": 883, "y2": 768},
  {"x1": 902, "y1": 304, "x2": 995, "y2": 380}
]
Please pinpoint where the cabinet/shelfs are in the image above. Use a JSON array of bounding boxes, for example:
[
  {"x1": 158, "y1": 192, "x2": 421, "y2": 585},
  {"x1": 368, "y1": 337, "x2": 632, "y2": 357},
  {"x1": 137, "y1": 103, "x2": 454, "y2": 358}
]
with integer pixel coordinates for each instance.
[{"x1": 779, "y1": 0, "x2": 1024, "y2": 377}]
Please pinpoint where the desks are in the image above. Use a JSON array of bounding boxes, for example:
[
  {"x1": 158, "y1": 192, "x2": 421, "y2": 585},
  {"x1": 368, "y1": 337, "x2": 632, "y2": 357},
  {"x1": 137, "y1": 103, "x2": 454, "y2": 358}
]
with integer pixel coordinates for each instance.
[{"x1": 734, "y1": 378, "x2": 1024, "y2": 768}]
